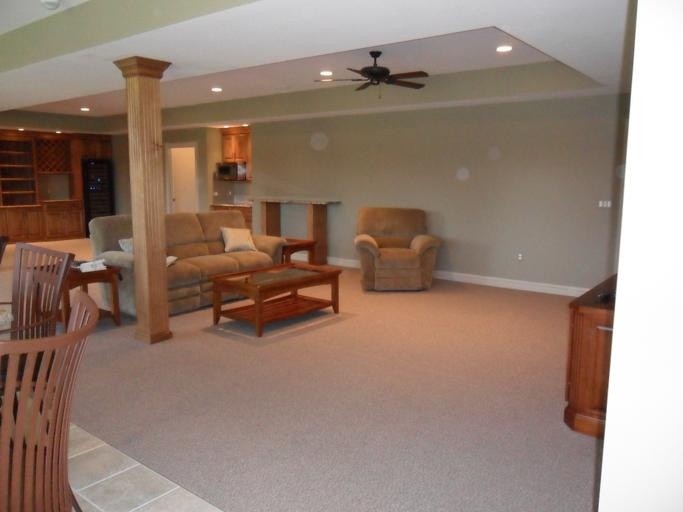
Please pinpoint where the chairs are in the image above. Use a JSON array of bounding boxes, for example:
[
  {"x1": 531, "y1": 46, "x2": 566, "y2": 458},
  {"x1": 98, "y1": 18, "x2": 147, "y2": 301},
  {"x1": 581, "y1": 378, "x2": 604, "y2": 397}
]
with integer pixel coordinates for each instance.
[
  {"x1": 352, "y1": 205, "x2": 438, "y2": 293},
  {"x1": 1, "y1": 241, "x2": 77, "y2": 392},
  {"x1": 0, "y1": 286, "x2": 102, "y2": 511}
]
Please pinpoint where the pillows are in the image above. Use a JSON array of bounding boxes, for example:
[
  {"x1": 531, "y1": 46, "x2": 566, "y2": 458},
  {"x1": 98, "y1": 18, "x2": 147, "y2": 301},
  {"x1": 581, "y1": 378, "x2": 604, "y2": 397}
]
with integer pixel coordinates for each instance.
[
  {"x1": 117, "y1": 235, "x2": 134, "y2": 254},
  {"x1": 218, "y1": 226, "x2": 255, "y2": 253}
]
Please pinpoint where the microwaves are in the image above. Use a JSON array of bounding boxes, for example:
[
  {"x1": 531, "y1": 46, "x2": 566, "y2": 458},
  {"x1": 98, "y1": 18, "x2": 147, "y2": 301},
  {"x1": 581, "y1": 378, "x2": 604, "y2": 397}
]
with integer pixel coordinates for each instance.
[{"x1": 215, "y1": 160, "x2": 246, "y2": 181}]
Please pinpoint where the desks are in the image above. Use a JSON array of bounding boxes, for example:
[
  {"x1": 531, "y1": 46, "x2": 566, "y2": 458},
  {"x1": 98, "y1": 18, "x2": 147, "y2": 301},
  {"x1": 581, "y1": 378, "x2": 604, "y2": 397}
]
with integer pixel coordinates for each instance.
[
  {"x1": 279, "y1": 236, "x2": 319, "y2": 266},
  {"x1": 27, "y1": 259, "x2": 120, "y2": 335}
]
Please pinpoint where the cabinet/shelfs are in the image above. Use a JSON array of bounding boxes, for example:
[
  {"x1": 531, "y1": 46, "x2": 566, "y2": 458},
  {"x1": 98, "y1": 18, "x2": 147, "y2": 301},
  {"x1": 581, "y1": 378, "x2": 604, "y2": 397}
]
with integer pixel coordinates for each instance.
[
  {"x1": 560, "y1": 272, "x2": 618, "y2": 437},
  {"x1": 70, "y1": 135, "x2": 112, "y2": 159},
  {"x1": 0, "y1": 136, "x2": 39, "y2": 206},
  {"x1": 41, "y1": 200, "x2": 81, "y2": 241},
  {"x1": 0, "y1": 204, "x2": 43, "y2": 244},
  {"x1": 209, "y1": 204, "x2": 251, "y2": 227},
  {"x1": 220, "y1": 125, "x2": 250, "y2": 165}
]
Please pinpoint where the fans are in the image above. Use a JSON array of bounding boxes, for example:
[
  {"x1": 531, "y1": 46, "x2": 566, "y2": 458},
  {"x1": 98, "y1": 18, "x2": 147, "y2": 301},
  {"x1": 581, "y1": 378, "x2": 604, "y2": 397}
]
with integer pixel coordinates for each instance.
[{"x1": 314, "y1": 50, "x2": 427, "y2": 93}]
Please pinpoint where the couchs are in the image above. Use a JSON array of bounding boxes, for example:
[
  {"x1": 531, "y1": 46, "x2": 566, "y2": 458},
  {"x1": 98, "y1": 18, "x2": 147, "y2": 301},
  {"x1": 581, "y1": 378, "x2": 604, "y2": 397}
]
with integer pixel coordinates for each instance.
[{"x1": 85, "y1": 209, "x2": 285, "y2": 320}]
